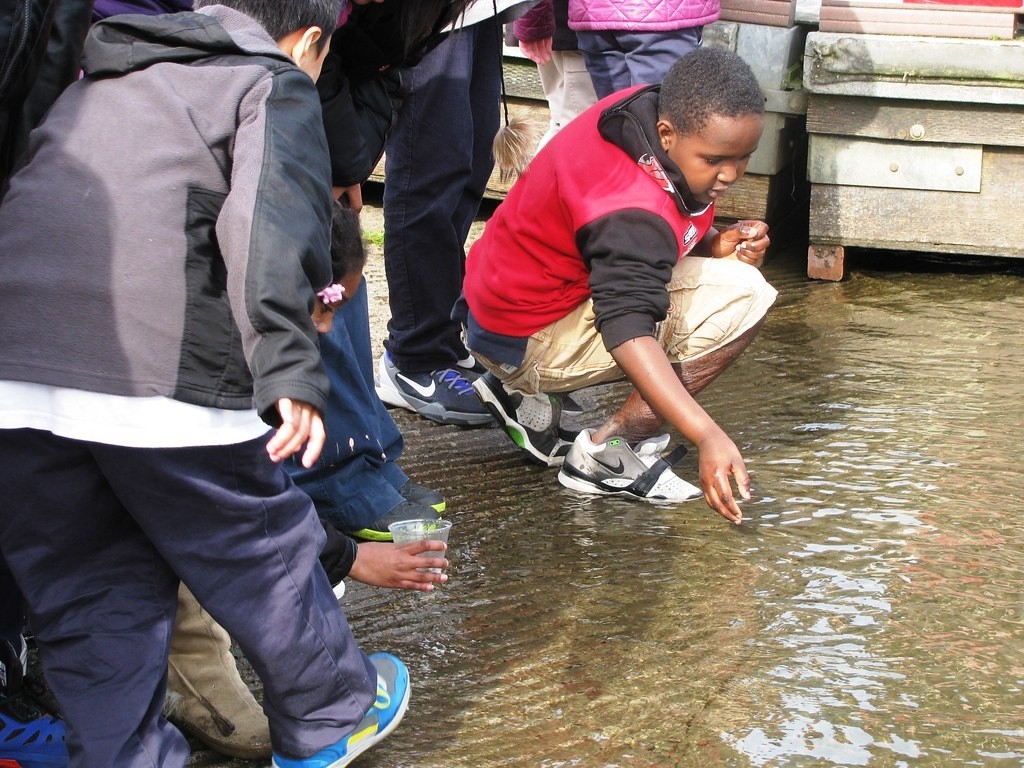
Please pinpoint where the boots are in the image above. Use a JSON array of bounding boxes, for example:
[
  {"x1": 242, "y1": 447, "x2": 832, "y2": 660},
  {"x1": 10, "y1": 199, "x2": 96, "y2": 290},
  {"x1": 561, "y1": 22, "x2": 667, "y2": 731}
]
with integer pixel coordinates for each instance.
[{"x1": 161, "y1": 581, "x2": 273, "y2": 758}]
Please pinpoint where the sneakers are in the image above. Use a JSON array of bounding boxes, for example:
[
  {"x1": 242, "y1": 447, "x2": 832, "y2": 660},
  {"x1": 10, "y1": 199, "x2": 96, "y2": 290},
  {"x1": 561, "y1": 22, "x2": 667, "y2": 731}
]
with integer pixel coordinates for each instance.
[
  {"x1": 345, "y1": 478, "x2": 446, "y2": 541},
  {"x1": 375, "y1": 348, "x2": 494, "y2": 425},
  {"x1": 472, "y1": 370, "x2": 581, "y2": 467},
  {"x1": 558, "y1": 429, "x2": 705, "y2": 505}
]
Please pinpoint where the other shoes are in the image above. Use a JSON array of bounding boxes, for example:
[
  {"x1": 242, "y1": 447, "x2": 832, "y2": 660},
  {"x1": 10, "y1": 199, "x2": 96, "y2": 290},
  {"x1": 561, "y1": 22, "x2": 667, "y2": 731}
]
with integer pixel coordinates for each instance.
[
  {"x1": 0, "y1": 690, "x2": 70, "y2": 768},
  {"x1": 272, "y1": 652, "x2": 410, "y2": 768}
]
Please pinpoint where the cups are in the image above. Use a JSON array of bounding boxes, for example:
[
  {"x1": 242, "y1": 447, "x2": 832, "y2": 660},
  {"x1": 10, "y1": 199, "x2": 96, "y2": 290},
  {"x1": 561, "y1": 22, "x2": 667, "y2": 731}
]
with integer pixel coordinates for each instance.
[{"x1": 388, "y1": 518, "x2": 453, "y2": 574}]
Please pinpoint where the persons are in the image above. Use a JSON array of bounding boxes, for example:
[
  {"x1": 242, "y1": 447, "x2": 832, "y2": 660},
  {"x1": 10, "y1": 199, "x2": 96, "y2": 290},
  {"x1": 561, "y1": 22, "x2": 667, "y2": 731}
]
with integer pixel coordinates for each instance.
[
  {"x1": 0, "y1": 0, "x2": 719, "y2": 768},
  {"x1": 459, "y1": 45, "x2": 782, "y2": 524}
]
